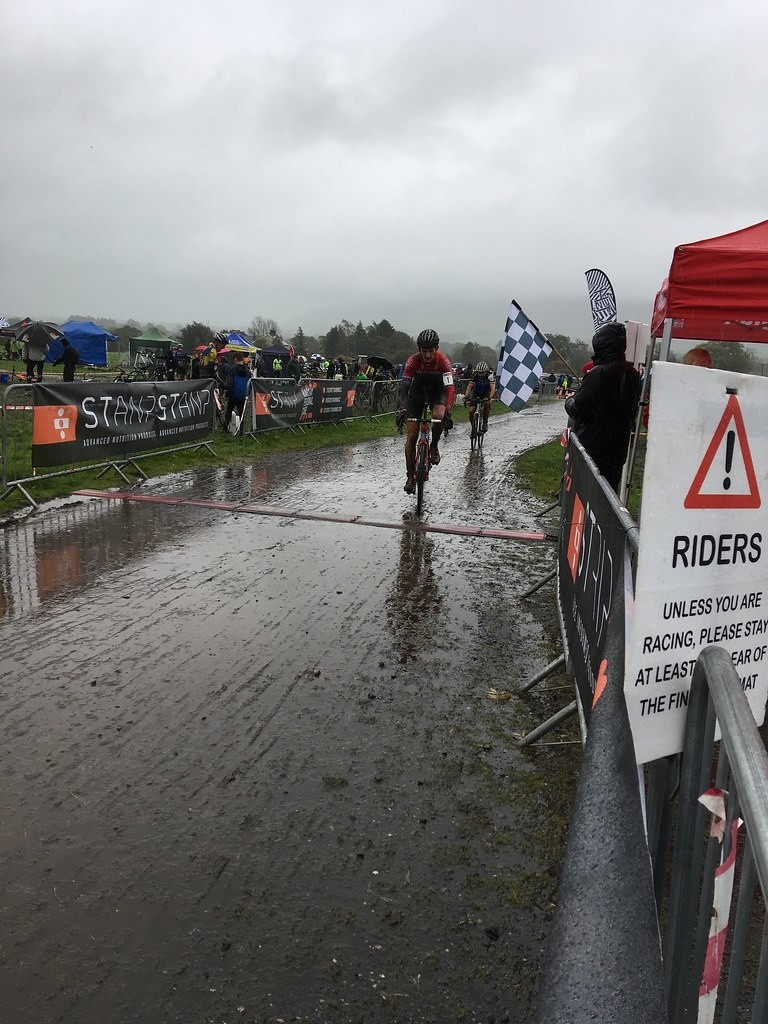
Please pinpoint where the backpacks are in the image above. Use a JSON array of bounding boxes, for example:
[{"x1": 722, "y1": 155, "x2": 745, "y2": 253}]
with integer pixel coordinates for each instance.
[{"x1": 67, "y1": 348, "x2": 79, "y2": 364}]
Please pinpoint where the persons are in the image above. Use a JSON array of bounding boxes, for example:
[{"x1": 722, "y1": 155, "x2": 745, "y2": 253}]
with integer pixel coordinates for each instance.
[
  {"x1": 564, "y1": 323, "x2": 643, "y2": 494},
  {"x1": 21, "y1": 343, "x2": 49, "y2": 384},
  {"x1": 165, "y1": 333, "x2": 584, "y2": 440},
  {"x1": 396, "y1": 329, "x2": 455, "y2": 494},
  {"x1": 52, "y1": 338, "x2": 81, "y2": 383},
  {"x1": 4, "y1": 338, "x2": 19, "y2": 361}
]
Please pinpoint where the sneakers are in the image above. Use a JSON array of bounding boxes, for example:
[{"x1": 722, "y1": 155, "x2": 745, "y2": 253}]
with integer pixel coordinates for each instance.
[
  {"x1": 404, "y1": 474, "x2": 415, "y2": 491},
  {"x1": 430, "y1": 448, "x2": 440, "y2": 464}
]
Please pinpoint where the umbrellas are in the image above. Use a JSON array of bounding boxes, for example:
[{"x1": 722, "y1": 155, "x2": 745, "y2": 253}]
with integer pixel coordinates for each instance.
[{"x1": 13, "y1": 320, "x2": 65, "y2": 344}]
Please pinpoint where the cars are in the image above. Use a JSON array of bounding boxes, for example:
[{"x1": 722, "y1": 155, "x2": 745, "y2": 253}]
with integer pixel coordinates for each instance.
[
  {"x1": 451, "y1": 363, "x2": 466, "y2": 375},
  {"x1": 534, "y1": 372, "x2": 583, "y2": 398}
]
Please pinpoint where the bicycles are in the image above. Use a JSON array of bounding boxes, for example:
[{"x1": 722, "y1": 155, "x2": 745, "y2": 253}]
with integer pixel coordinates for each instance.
[
  {"x1": 81, "y1": 348, "x2": 353, "y2": 434},
  {"x1": 10, "y1": 366, "x2": 39, "y2": 389},
  {"x1": 396, "y1": 416, "x2": 450, "y2": 517},
  {"x1": 353, "y1": 379, "x2": 400, "y2": 415},
  {"x1": 463, "y1": 396, "x2": 494, "y2": 451}
]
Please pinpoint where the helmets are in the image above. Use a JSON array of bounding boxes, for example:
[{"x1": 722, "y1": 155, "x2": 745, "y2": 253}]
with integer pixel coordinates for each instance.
[
  {"x1": 476, "y1": 362, "x2": 489, "y2": 372},
  {"x1": 417, "y1": 329, "x2": 439, "y2": 349},
  {"x1": 213, "y1": 333, "x2": 229, "y2": 343}
]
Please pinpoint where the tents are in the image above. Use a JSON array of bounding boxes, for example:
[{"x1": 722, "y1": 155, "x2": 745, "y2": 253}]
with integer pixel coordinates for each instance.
[
  {"x1": 0, "y1": 317, "x2": 37, "y2": 361},
  {"x1": 129, "y1": 327, "x2": 180, "y2": 368},
  {"x1": 44, "y1": 320, "x2": 121, "y2": 367},
  {"x1": 621, "y1": 219, "x2": 768, "y2": 507}
]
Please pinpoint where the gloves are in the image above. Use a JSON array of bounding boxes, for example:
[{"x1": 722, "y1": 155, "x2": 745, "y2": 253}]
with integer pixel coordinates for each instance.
[
  {"x1": 395, "y1": 413, "x2": 407, "y2": 427},
  {"x1": 443, "y1": 414, "x2": 453, "y2": 434}
]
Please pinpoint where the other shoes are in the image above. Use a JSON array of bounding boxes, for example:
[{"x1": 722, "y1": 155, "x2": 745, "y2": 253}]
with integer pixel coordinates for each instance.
[{"x1": 482, "y1": 423, "x2": 488, "y2": 432}]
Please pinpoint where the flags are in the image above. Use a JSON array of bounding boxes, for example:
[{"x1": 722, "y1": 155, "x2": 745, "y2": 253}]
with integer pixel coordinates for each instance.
[{"x1": 495, "y1": 299, "x2": 556, "y2": 413}]
